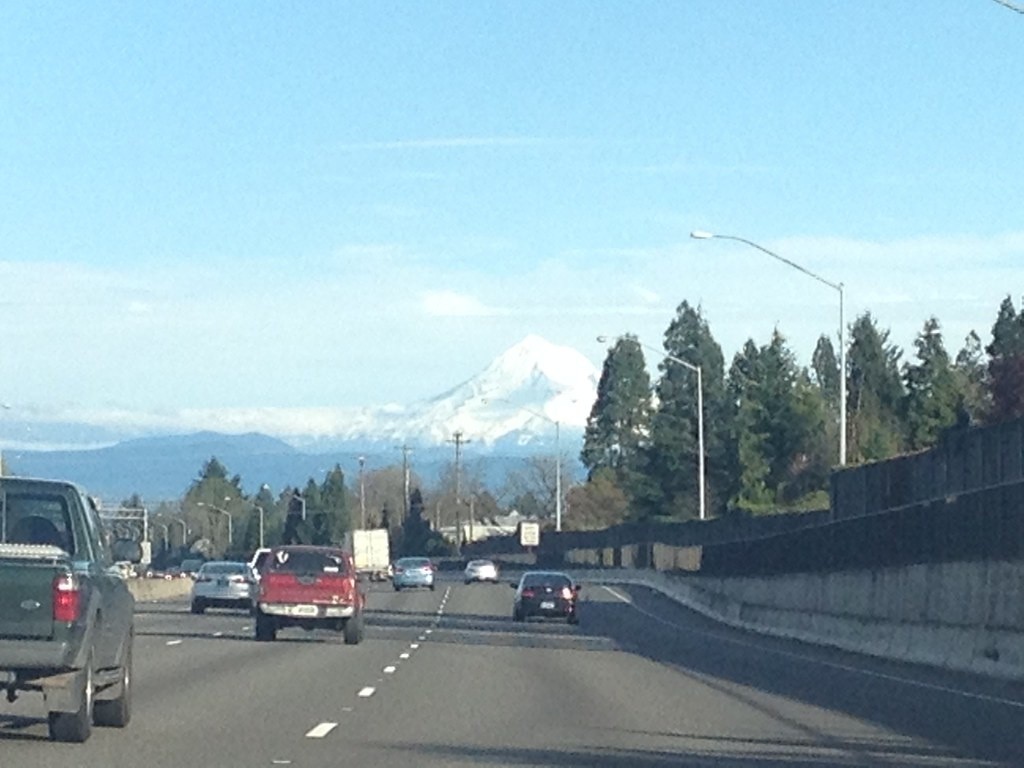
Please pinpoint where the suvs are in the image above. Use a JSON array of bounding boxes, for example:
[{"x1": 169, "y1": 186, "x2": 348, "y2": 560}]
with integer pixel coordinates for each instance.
[{"x1": 0, "y1": 475, "x2": 140, "y2": 746}]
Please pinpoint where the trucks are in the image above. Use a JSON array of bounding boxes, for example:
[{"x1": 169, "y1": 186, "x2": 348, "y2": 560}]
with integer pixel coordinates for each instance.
[{"x1": 348, "y1": 527, "x2": 392, "y2": 583}]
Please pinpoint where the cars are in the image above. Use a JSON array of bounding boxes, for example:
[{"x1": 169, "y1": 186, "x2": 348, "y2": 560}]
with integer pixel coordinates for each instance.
[
  {"x1": 509, "y1": 570, "x2": 583, "y2": 627},
  {"x1": 251, "y1": 543, "x2": 366, "y2": 646},
  {"x1": 390, "y1": 556, "x2": 438, "y2": 592},
  {"x1": 189, "y1": 559, "x2": 255, "y2": 617},
  {"x1": 461, "y1": 558, "x2": 502, "y2": 586}
]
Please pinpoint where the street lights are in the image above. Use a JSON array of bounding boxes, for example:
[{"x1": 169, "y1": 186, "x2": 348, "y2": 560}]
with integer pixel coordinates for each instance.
[
  {"x1": 691, "y1": 229, "x2": 849, "y2": 469},
  {"x1": 223, "y1": 495, "x2": 264, "y2": 548},
  {"x1": 597, "y1": 332, "x2": 706, "y2": 521},
  {"x1": 196, "y1": 501, "x2": 233, "y2": 545},
  {"x1": 480, "y1": 397, "x2": 563, "y2": 533},
  {"x1": 261, "y1": 484, "x2": 308, "y2": 523},
  {"x1": 157, "y1": 513, "x2": 187, "y2": 546}
]
